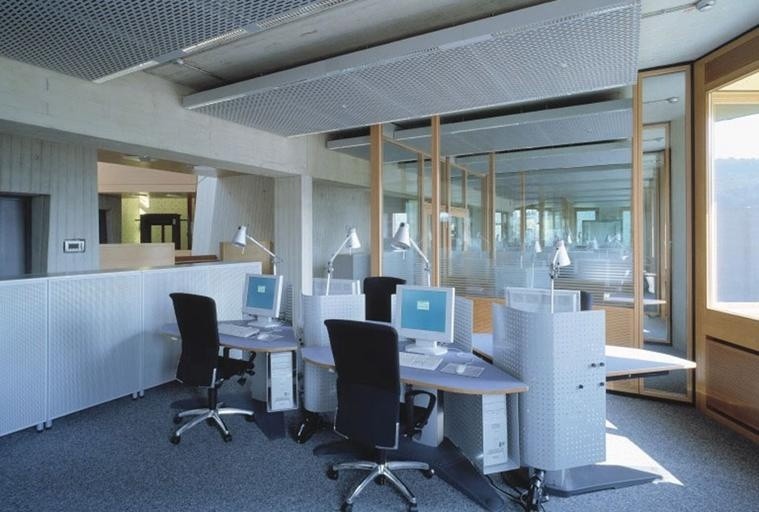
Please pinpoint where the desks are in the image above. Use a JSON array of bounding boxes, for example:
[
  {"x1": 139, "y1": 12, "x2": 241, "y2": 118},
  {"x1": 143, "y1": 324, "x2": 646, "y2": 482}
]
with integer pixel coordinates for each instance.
[
  {"x1": 467, "y1": 331, "x2": 697, "y2": 499},
  {"x1": 301, "y1": 336, "x2": 531, "y2": 477},
  {"x1": 161, "y1": 320, "x2": 300, "y2": 414}
]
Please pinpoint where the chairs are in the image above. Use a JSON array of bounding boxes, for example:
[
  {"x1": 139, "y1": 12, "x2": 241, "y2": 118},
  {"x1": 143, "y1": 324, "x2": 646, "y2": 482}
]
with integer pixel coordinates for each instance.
[
  {"x1": 167, "y1": 291, "x2": 257, "y2": 444},
  {"x1": 323, "y1": 320, "x2": 435, "y2": 511},
  {"x1": 363, "y1": 276, "x2": 408, "y2": 323}
]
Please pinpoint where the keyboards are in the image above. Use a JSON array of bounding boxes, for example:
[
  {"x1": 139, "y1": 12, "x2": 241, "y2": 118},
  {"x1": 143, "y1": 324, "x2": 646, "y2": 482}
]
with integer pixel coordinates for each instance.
[
  {"x1": 398, "y1": 352, "x2": 444, "y2": 370},
  {"x1": 218, "y1": 323, "x2": 260, "y2": 337}
]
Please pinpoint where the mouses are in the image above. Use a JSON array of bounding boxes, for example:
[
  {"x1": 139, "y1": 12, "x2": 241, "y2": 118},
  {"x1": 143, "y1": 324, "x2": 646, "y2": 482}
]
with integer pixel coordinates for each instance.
[
  {"x1": 455, "y1": 364, "x2": 466, "y2": 374},
  {"x1": 257, "y1": 333, "x2": 269, "y2": 339}
]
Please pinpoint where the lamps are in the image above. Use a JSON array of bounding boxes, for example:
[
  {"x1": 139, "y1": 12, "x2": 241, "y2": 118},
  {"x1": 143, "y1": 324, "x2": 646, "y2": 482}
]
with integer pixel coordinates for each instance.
[
  {"x1": 546, "y1": 239, "x2": 572, "y2": 314},
  {"x1": 324, "y1": 221, "x2": 432, "y2": 295},
  {"x1": 230, "y1": 223, "x2": 283, "y2": 276}
]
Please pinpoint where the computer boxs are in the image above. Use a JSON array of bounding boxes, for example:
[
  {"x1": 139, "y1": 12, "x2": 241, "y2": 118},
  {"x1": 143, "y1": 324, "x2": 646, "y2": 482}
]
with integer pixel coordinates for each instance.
[
  {"x1": 270, "y1": 352, "x2": 293, "y2": 410},
  {"x1": 482, "y1": 394, "x2": 509, "y2": 467}
]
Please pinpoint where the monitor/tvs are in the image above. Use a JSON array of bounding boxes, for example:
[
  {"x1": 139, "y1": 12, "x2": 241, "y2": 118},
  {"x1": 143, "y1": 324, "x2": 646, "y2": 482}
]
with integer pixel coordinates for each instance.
[
  {"x1": 506, "y1": 287, "x2": 581, "y2": 314},
  {"x1": 242, "y1": 274, "x2": 283, "y2": 327},
  {"x1": 313, "y1": 278, "x2": 361, "y2": 295},
  {"x1": 395, "y1": 285, "x2": 455, "y2": 356}
]
